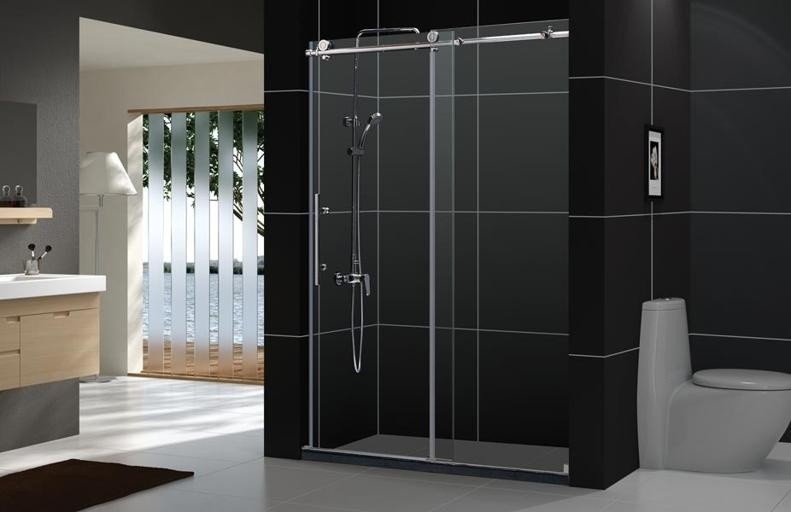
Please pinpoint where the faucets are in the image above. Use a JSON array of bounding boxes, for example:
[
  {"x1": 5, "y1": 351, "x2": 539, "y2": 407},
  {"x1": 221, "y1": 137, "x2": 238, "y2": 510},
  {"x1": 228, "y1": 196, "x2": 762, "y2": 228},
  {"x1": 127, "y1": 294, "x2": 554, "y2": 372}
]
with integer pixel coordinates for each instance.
[{"x1": 22, "y1": 243, "x2": 53, "y2": 276}]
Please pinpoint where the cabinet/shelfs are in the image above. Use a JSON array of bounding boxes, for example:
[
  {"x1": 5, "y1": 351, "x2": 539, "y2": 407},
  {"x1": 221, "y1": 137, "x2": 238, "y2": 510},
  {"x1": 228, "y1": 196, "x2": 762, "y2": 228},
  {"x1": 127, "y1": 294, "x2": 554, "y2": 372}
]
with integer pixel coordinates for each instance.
[{"x1": 1, "y1": 272, "x2": 107, "y2": 452}]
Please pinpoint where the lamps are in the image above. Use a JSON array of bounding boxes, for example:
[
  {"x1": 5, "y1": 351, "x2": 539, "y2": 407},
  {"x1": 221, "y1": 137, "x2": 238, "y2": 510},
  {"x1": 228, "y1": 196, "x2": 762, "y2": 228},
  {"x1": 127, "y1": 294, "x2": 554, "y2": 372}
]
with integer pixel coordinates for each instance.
[{"x1": 79, "y1": 150, "x2": 139, "y2": 382}]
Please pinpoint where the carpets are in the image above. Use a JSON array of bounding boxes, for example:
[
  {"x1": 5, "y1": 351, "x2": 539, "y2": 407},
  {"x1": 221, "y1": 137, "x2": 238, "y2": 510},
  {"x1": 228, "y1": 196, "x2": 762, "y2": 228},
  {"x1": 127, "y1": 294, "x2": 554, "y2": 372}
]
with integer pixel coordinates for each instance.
[{"x1": 0, "y1": 458, "x2": 195, "y2": 510}]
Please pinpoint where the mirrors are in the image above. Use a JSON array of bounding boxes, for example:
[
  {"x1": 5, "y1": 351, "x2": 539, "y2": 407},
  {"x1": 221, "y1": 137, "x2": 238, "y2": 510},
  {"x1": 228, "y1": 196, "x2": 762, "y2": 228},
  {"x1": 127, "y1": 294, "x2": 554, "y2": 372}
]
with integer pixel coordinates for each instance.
[{"x1": 0, "y1": 101, "x2": 38, "y2": 205}]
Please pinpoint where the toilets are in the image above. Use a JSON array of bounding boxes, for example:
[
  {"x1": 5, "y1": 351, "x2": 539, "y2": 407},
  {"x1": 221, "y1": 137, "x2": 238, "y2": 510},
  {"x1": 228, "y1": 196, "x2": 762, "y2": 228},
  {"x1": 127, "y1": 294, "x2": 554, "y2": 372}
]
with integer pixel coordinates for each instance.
[{"x1": 636, "y1": 296, "x2": 791, "y2": 473}]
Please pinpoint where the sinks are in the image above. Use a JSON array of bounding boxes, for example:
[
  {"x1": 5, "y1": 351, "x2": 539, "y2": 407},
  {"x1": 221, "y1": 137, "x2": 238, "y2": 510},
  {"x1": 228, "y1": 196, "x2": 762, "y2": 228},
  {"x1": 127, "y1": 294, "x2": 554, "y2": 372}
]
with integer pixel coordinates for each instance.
[{"x1": 0, "y1": 272, "x2": 62, "y2": 281}]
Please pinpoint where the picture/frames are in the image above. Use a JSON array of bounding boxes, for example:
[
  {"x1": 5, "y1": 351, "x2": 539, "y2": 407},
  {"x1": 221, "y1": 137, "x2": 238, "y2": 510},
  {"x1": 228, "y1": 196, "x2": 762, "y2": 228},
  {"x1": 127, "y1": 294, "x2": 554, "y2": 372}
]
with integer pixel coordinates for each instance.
[{"x1": 644, "y1": 123, "x2": 666, "y2": 200}]
